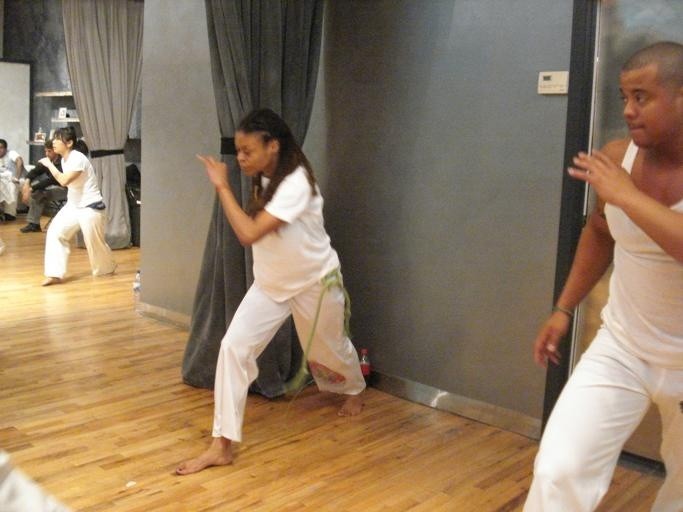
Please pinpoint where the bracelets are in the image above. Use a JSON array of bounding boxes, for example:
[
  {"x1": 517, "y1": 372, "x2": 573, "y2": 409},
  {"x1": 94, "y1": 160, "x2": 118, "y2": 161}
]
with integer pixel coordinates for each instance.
[
  {"x1": 551, "y1": 304, "x2": 572, "y2": 317},
  {"x1": 211, "y1": 185, "x2": 231, "y2": 192}
]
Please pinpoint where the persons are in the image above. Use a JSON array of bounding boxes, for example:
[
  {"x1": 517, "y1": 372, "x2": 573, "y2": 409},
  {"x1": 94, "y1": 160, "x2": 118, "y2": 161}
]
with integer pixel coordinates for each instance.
[
  {"x1": 170, "y1": 109, "x2": 366, "y2": 478},
  {"x1": 0, "y1": 139, "x2": 34, "y2": 220},
  {"x1": 18, "y1": 141, "x2": 71, "y2": 233},
  {"x1": 516, "y1": 41, "x2": 683, "y2": 512},
  {"x1": 37, "y1": 125, "x2": 119, "y2": 287}
]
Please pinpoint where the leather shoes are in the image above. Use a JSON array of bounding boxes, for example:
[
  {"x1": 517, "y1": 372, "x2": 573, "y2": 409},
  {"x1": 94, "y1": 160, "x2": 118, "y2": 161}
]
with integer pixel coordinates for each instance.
[{"x1": 19, "y1": 223, "x2": 41, "y2": 233}]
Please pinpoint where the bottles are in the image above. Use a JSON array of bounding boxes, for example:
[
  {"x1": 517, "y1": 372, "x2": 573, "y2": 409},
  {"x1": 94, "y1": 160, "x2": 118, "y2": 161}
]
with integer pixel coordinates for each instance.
[
  {"x1": 133, "y1": 270, "x2": 140, "y2": 296},
  {"x1": 359, "y1": 348, "x2": 370, "y2": 387}
]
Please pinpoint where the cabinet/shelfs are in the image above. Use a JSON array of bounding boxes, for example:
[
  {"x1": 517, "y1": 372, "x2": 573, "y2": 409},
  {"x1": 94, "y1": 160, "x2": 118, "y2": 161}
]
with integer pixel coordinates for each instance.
[{"x1": 25, "y1": 90, "x2": 80, "y2": 146}]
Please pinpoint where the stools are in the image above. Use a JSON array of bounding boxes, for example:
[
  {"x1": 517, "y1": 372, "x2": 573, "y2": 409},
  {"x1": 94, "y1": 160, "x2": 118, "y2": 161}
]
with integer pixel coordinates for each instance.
[{"x1": 45, "y1": 199, "x2": 68, "y2": 228}]
